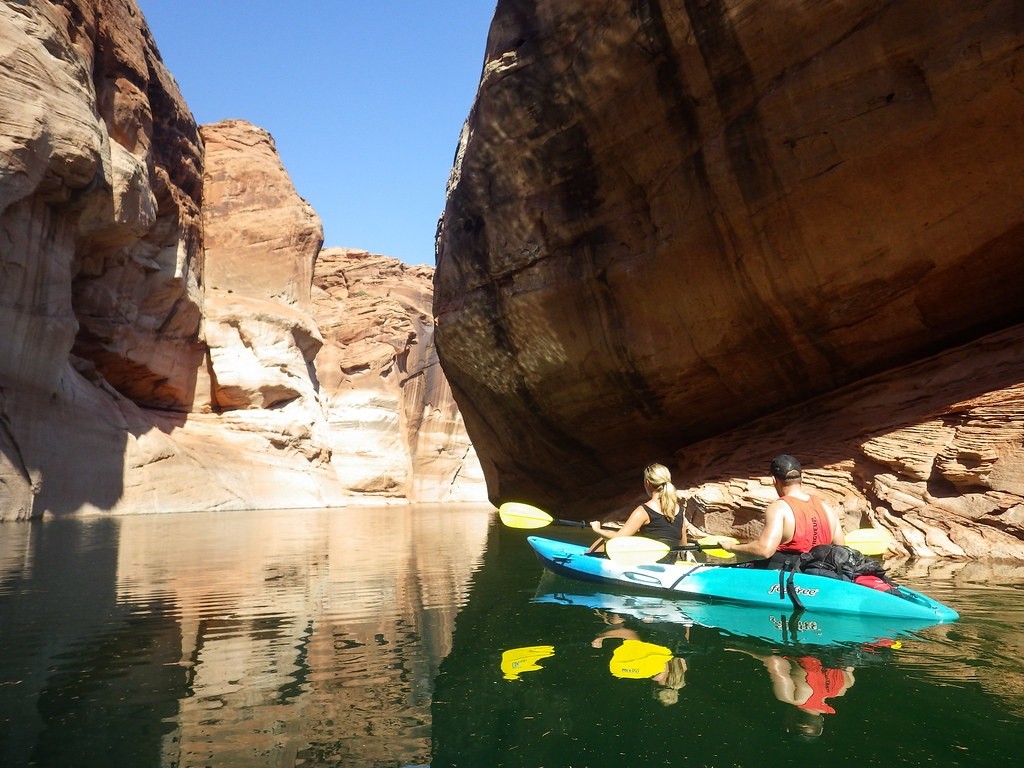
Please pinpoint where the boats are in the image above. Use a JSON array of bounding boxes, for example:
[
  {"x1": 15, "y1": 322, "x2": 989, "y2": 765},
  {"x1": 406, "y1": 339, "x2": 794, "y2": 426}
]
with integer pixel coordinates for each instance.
[
  {"x1": 528, "y1": 567, "x2": 938, "y2": 650},
  {"x1": 527, "y1": 536, "x2": 961, "y2": 625}
]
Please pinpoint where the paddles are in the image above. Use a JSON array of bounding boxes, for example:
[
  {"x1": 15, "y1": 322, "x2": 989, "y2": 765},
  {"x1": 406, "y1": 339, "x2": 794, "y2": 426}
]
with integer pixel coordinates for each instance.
[
  {"x1": 604, "y1": 527, "x2": 894, "y2": 564},
  {"x1": 497, "y1": 500, "x2": 742, "y2": 560},
  {"x1": 608, "y1": 638, "x2": 718, "y2": 681}
]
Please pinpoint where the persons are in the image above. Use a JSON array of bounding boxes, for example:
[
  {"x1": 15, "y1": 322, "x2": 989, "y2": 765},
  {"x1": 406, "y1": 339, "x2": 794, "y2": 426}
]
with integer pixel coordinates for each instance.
[
  {"x1": 722, "y1": 636, "x2": 855, "y2": 749},
  {"x1": 717, "y1": 453, "x2": 845, "y2": 565},
  {"x1": 587, "y1": 463, "x2": 689, "y2": 565},
  {"x1": 589, "y1": 610, "x2": 692, "y2": 710}
]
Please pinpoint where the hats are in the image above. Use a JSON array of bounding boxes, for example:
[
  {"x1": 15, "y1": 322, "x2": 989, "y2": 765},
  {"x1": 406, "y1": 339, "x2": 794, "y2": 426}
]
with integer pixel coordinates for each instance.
[{"x1": 768, "y1": 454, "x2": 802, "y2": 480}]
